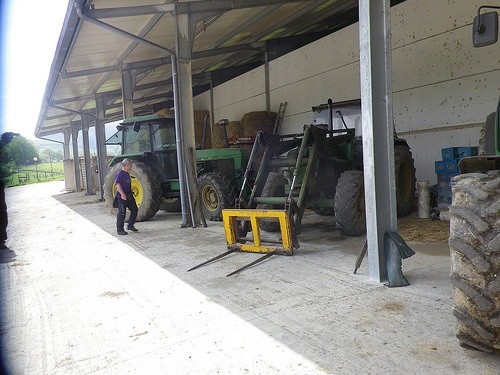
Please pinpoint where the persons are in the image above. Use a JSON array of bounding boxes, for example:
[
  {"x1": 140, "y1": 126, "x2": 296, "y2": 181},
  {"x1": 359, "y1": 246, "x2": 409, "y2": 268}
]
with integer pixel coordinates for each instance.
[{"x1": 115, "y1": 158, "x2": 139, "y2": 236}]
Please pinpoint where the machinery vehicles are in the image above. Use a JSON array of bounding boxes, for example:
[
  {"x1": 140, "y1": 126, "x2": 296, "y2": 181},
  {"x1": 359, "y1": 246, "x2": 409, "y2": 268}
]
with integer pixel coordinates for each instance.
[
  {"x1": 448, "y1": 5, "x2": 500, "y2": 355},
  {"x1": 103, "y1": 112, "x2": 250, "y2": 222},
  {"x1": 187, "y1": 97, "x2": 417, "y2": 278}
]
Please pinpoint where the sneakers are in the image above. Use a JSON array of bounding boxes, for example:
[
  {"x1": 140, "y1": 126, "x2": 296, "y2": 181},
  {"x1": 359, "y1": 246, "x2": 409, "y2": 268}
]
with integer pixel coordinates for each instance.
[
  {"x1": 117, "y1": 231, "x2": 128, "y2": 234},
  {"x1": 128, "y1": 226, "x2": 138, "y2": 230}
]
